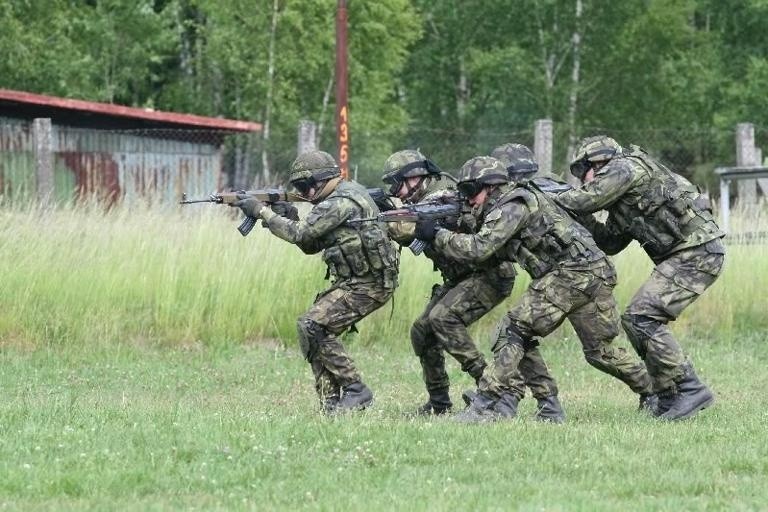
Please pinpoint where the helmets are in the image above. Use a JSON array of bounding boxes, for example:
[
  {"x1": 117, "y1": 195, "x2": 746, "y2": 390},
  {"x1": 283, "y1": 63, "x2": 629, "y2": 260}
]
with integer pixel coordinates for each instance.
[
  {"x1": 457, "y1": 156, "x2": 510, "y2": 184},
  {"x1": 290, "y1": 152, "x2": 342, "y2": 182},
  {"x1": 381, "y1": 149, "x2": 437, "y2": 179},
  {"x1": 573, "y1": 136, "x2": 623, "y2": 162},
  {"x1": 490, "y1": 142, "x2": 539, "y2": 173}
]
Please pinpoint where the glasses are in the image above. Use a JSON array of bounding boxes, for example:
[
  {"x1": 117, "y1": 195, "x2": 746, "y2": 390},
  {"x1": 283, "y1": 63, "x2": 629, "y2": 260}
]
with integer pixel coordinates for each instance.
[
  {"x1": 292, "y1": 183, "x2": 311, "y2": 196},
  {"x1": 454, "y1": 181, "x2": 483, "y2": 199},
  {"x1": 390, "y1": 176, "x2": 404, "y2": 194},
  {"x1": 569, "y1": 162, "x2": 590, "y2": 177}
]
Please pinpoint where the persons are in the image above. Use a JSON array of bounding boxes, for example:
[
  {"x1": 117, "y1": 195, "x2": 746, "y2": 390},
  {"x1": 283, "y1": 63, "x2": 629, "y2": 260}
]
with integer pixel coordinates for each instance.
[
  {"x1": 462, "y1": 143, "x2": 667, "y2": 414},
  {"x1": 226, "y1": 150, "x2": 401, "y2": 415},
  {"x1": 542, "y1": 135, "x2": 724, "y2": 421},
  {"x1": 382, "y1": 149, "x2": 516, "y2": 417},
  {"x1": 415, "y1": 156, "x2": 606, "y2": 418}
]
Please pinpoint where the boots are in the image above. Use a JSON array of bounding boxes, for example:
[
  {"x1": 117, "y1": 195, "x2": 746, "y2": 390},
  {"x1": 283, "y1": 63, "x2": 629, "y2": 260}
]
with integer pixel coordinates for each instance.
[
  {"x1": 333, "y1": 382, "x2": 373, "y2": 411},
  {"x1": 636, "y1": 391, "x2": 654, "y2": 415},
  {"x1": 651, "y1": 386, "x2": 679, "y2": 416},
  {"x1": 462, "y1": 390, "x2": 518, "y2": 420},
  {"x1": 658, "y1": 372, "x2": 713, "y2": 420},
  {"x1": 531, "y1": 396, "x2": 566, "y2": 423},
  {"x1": 448, "y1": 390, "x2": 498, "y2": 423},
  {"x1": 402, "y1": 393, "x2": 453, "y2": 416},
  {"x1": 318, "y1": 394, "x2": 340, "y2": 412}
]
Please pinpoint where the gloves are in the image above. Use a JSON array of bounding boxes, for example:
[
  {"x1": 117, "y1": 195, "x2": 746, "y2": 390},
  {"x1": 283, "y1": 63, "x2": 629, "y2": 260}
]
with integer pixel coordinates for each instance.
[
  {"x1": 270, "y1": 200, "x2": 299, "y2": 222},
  {"x1": 230, "y1": 194, "x2": 264, "y2": 219},
  {"x1": 414, "y1": 213, "x2": 436, "y2": 241}
]
